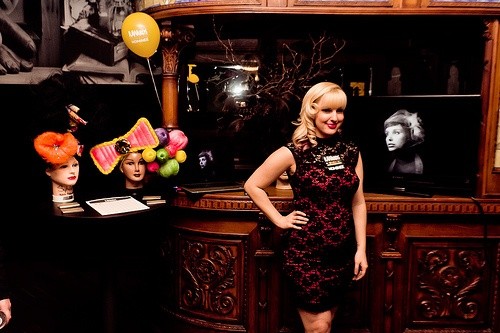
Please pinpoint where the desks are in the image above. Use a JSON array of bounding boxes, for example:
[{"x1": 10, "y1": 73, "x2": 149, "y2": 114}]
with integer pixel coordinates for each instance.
[{"x1": 52, "y1": 195, "x2": 167, "y2": 333}]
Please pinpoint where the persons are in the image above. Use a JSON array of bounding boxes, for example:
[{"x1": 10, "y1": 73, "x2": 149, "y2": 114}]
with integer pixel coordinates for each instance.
[
  {"x1": 198, "y1": 150, "x2": 216, "y2": 168},
  {"x1": 89, "y1": 118, "x2": 188, "y2": 187},
  {"x1": 0, "y1": 86, "x2": 20, "y2": 333},
  {"x1": 371, "y1": 109, "x2": 437, "y2": 194},
  {"x1": 276, "y1": 170, "x2": 293, "y2": 190},
  {"x1": 243, "y1": 83, "x2": 368, "y2": 333},
  {"x1": 34, "y1": 132, "x2": 84, "y2": 202}
]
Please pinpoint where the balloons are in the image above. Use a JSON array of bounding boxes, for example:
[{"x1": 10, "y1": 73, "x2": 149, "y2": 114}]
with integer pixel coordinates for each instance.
[{"x1": 121, "y1": 11, "x2": 162, "y2": 58}]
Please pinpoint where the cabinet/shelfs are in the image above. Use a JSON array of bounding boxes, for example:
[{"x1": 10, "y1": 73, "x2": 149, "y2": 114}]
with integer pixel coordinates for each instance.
[{"x1": 173, "y1": 181, "x2": 500, "y2": 333}]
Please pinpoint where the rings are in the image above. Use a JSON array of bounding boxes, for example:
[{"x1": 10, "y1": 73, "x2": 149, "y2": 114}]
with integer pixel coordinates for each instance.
[{"x1": 359, "y1": 270, "x2": 363, "y2": 274}]
